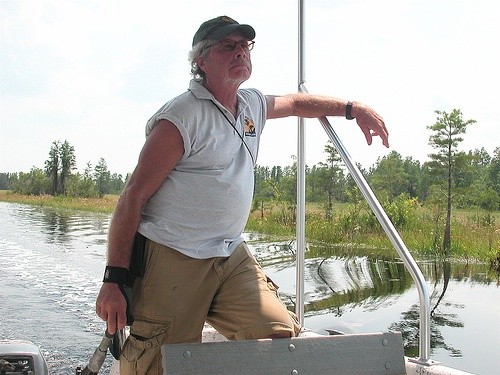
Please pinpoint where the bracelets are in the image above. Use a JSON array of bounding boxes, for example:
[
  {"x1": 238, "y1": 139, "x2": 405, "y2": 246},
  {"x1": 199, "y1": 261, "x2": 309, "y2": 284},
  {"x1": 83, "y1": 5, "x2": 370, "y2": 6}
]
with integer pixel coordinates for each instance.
[
  {"x1": 345, "y1": 101, "x2": 355, "y2": 120},
  {"x1": 103, "y1": 266, "x2": 130, "y2": 286}
]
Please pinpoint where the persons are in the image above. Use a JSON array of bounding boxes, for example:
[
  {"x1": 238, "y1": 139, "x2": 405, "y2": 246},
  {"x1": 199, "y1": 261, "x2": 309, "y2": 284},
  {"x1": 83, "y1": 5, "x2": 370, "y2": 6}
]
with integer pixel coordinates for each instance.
[{"x1": 96, "y1": 16, "x2": 389, "y2": 375}]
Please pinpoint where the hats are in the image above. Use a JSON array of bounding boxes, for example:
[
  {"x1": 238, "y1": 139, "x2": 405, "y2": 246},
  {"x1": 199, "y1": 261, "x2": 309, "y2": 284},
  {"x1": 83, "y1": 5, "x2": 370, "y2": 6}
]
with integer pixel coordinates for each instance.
[{"x1": 192, "y1": 16, "x2": 256, "y2": 45}]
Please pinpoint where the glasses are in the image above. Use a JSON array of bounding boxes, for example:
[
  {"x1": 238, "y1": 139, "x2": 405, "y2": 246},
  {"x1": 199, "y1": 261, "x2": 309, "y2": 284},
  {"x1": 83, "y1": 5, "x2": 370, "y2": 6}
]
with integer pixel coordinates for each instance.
[{"x1": 205, "y1": 39, "x2": 255, "y2": 51}]
